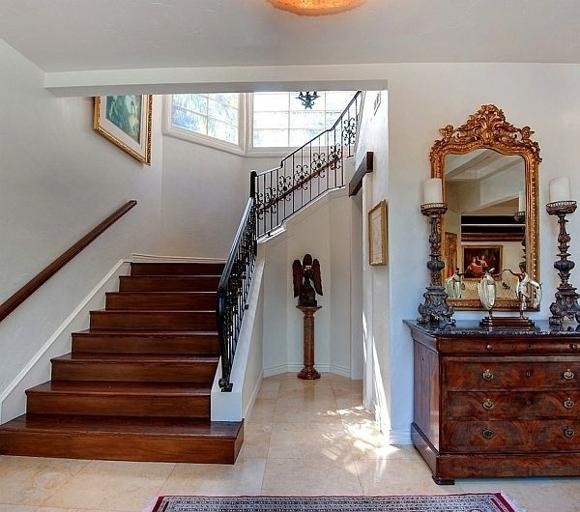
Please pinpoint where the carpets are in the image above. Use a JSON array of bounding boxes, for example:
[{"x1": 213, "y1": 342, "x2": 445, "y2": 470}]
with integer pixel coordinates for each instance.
[{"x1": 144, "y1": 488, "x2": 527, "y2": 512}]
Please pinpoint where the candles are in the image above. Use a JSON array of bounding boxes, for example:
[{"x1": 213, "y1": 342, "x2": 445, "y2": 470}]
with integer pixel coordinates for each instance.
[
  {"x1": 423, "y1": 177, "x2": 443, "y2": 207},
  {"x1": 519, "y1": 190, "x2": 525, "y2": 210},
  {"x1": 549, "y1": 175, "x2": 572, "y2": 202}
]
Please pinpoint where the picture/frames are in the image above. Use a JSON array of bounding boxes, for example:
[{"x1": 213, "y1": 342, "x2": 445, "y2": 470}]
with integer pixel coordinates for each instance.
[
  {"x1": 93, "y1": 94, "x2": 152, "y2": 166},
  {"x1": 462, "y1": 244, "x2": 503, "y2": 282},
  {"x1": 368, "y1": 199, "x2": 388, "y2": 266}
]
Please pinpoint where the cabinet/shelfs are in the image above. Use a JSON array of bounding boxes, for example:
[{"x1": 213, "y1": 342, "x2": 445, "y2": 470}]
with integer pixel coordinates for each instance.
[{"x1": 403, "y1": 320, "x2": 580, "y2": 485}]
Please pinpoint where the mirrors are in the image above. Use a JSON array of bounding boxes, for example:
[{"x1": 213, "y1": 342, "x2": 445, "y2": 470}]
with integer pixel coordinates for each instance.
[{"x1": 429, "y1": 104, "x2": 542, "y2": 310}]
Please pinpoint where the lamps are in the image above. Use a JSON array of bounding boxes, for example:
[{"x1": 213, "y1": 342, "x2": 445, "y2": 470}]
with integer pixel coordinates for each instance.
[{"x1": 266, "y1": 0, "x2": 368, "y2": 16}]
